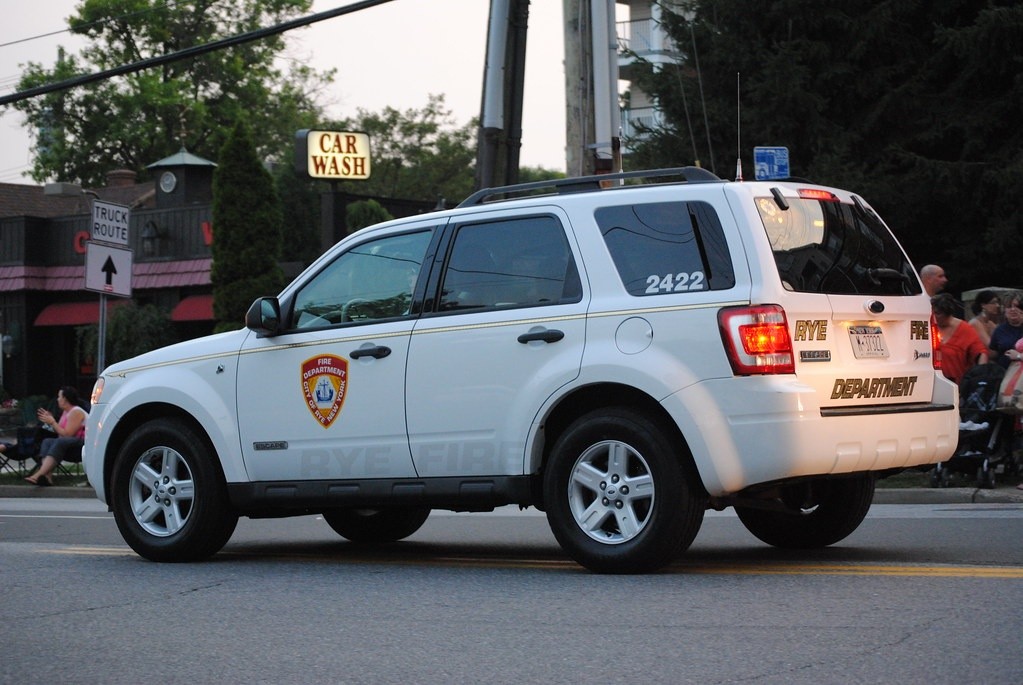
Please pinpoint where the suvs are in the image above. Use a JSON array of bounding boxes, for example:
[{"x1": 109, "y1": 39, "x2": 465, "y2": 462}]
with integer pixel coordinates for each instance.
[{"x1": 81, "y1": 167, "x2": 961, "y2": 574}]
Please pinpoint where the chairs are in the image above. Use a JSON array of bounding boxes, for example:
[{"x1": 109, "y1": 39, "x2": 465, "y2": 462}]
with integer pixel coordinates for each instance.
[
  {"x1": 0, "y1": 427, "x2": 45, "y2": 480},
  {"x1": 56, "y1": 446, "x2": 82, "y2": 482}
]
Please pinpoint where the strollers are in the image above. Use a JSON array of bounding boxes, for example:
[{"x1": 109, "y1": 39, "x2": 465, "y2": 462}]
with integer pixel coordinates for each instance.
[{"x1": 930, "y1": 362, "x2": 1015, "y2": 489}]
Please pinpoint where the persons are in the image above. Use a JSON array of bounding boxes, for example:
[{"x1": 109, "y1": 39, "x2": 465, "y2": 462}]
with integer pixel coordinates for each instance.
[
  {"x1": 24, "y1": 386, "x2": 89, "y2": 487},
  {"x1": 920, "y1": 265, "x2": 1023, "y2": 386}
]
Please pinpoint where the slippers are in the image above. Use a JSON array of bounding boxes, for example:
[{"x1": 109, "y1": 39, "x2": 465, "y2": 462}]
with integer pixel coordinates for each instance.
[
  {"x1": 44, "y1": 475, "x2": 53, "y2": 486},
  {"x1": 24, "y1": 477, "x2": 38, "y2": 485}
]
